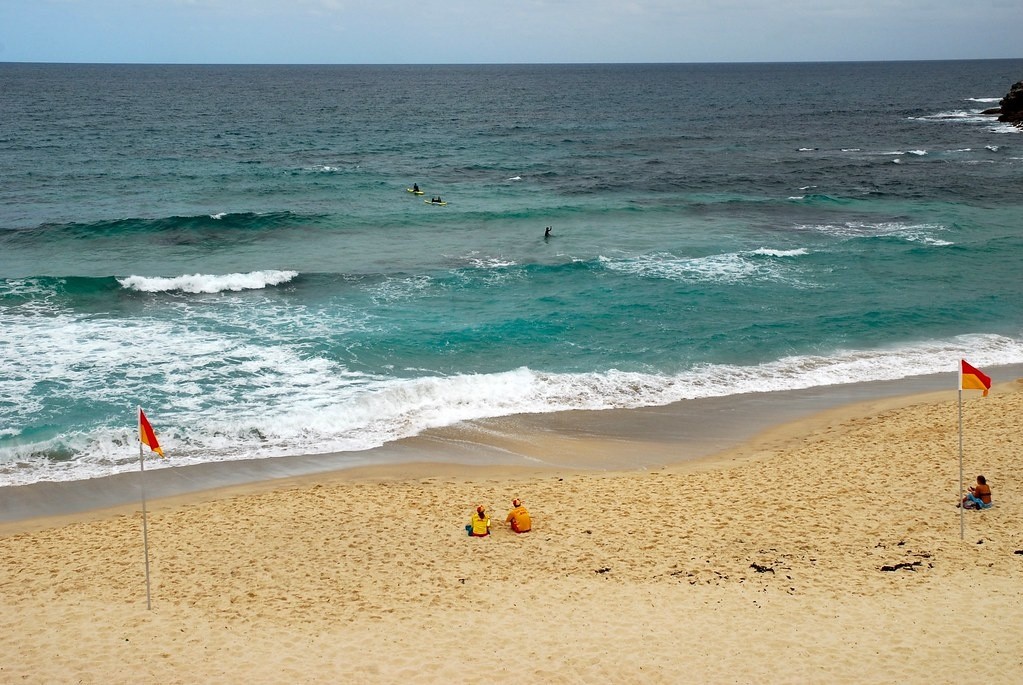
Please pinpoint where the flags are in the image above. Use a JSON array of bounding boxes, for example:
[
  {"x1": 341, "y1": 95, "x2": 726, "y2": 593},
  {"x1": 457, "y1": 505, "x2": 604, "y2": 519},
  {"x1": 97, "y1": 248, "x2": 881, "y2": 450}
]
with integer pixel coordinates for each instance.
[
  {"x1": 959, "y1": 359, "x2": 993, "y2": 398},
  {"x1": 139, "y1": 409, "x2": 167, "y2": 458}
]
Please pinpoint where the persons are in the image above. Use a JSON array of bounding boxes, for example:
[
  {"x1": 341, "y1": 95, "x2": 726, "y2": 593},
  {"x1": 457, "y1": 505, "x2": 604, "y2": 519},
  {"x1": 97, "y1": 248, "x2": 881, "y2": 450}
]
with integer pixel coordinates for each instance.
[
  {"x1": 413, "y1": 183, "x2": 419, "y2": 192},
  {"x1": 505, "y1": 498, "x2": 532, "y2": 533},
  {"x1": 432, "y1": 196, "x2": 442, "y2": 203},
  {"x1": 955, "y1": 475, "x2": 992, "y2": 508},
  {"x1": 470, "y1": 506, "x2": 490, "y2": 537},
  {"x1": 544, "y1": 225, "x2": 553, "y2": 236}
]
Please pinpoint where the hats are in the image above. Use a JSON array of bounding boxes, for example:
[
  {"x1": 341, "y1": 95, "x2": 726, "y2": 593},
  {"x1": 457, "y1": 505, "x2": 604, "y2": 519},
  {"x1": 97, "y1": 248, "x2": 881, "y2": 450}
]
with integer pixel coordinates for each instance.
[
  {"x1": 477, "y1": 505, "x2": 485, "y2": 513},
  {"x1": 513, "y1": 499, "x2": 520, "y2": 506}
]
